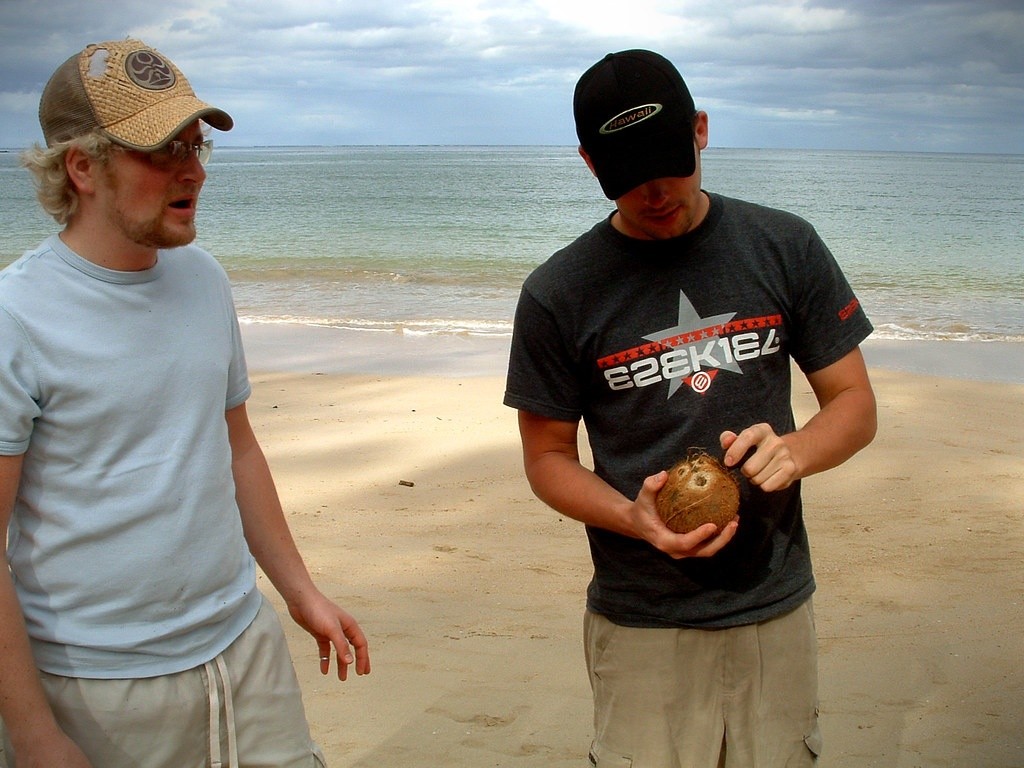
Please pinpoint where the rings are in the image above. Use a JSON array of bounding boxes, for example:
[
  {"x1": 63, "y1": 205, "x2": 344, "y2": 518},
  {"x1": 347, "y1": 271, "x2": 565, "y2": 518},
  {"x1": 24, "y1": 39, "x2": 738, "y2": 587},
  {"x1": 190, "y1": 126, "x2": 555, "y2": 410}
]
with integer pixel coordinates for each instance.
[{"x1": 320, "y1": 656, "x2": 329, "y2": 660}]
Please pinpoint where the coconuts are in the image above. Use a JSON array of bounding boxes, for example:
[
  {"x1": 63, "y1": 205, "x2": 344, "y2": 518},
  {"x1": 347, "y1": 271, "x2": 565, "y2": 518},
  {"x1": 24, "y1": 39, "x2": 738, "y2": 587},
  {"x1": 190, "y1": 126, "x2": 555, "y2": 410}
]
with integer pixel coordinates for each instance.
[{"x1": 655, "y1": 452, "x2": 740, "y2": 540}]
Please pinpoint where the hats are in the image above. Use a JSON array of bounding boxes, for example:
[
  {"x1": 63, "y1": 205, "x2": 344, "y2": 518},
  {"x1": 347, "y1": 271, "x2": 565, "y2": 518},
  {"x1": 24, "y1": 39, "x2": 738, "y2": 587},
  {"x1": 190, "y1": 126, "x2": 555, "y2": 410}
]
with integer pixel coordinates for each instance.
[
  {"x1": 38, "y1": 39, "x2": 234, "y2": 149},
  {"x1": 574, "y1": 50, "x2": 697, "y2": 200}
]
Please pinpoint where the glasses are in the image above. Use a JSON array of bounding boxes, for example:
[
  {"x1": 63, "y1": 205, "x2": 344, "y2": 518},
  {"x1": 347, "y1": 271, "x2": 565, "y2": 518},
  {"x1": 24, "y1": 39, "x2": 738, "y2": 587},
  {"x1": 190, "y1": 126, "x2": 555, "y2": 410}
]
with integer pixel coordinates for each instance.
[{"x1": 99, "y1": 140, "x2": 212, "y2": 170}]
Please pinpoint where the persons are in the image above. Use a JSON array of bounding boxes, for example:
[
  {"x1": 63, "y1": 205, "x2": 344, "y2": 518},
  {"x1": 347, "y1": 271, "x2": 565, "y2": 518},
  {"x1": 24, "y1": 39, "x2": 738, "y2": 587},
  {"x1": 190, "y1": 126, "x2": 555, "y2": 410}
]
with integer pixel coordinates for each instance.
[
  {"x1": 0, "y1": 39, "x2": 373, "y2": 768},
  {"x1": 502, "y1": 48, "x2": 880, "y2": 768}
]
